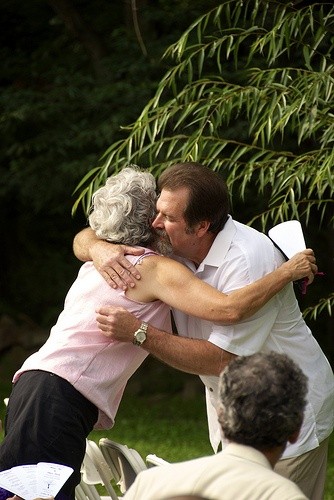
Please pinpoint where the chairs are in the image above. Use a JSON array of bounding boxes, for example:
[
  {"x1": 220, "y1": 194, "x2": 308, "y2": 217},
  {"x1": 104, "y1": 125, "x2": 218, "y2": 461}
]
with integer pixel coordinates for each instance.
[{"x1": 74, "y1": 438, "x2": 171, "y2": 500}]
